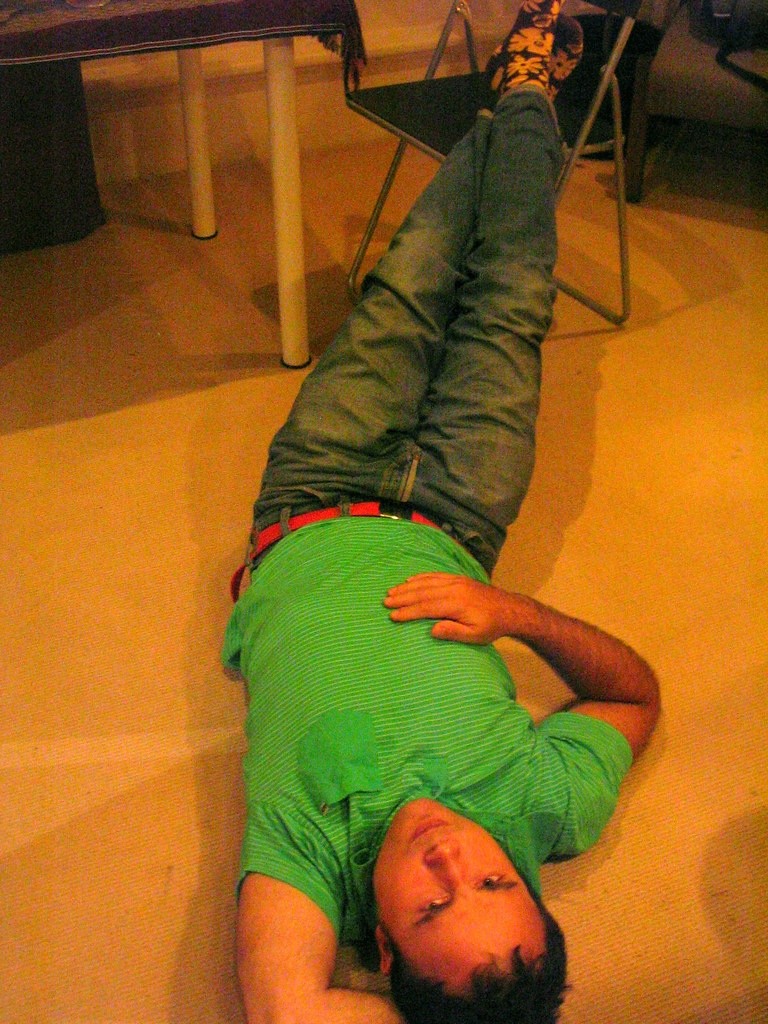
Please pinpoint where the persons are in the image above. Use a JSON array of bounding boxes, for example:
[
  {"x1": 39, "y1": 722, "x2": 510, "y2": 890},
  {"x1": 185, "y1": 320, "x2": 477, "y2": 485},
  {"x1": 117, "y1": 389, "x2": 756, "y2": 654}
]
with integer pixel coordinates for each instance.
[{"x1": 220, "y1": 0, "x2": 663, "y2": 1024}]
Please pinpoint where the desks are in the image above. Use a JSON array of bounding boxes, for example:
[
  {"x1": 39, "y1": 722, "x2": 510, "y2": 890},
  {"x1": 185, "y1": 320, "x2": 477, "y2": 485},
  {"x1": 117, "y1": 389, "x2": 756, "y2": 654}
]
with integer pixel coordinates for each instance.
[{"x1": 0, "y1": 0, "x2": 370, "y2": 370}]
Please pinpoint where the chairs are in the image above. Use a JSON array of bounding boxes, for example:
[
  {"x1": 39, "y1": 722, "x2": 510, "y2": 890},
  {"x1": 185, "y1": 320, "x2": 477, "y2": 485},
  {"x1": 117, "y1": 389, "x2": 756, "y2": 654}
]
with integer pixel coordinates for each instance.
[
  {"x1": 348, "y1": 0, "x2": 643, "y2": 329},
  {"x1": 624, "y1": 0, "x2": 768, "y2": 205}
]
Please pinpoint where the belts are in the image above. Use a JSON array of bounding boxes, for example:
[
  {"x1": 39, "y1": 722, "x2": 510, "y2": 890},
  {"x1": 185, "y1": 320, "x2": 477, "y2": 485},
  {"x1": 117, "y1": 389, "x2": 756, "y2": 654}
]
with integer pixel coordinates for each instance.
[{"x1": 231, "y1": 499, "x2": 444, "y2": 602}]
